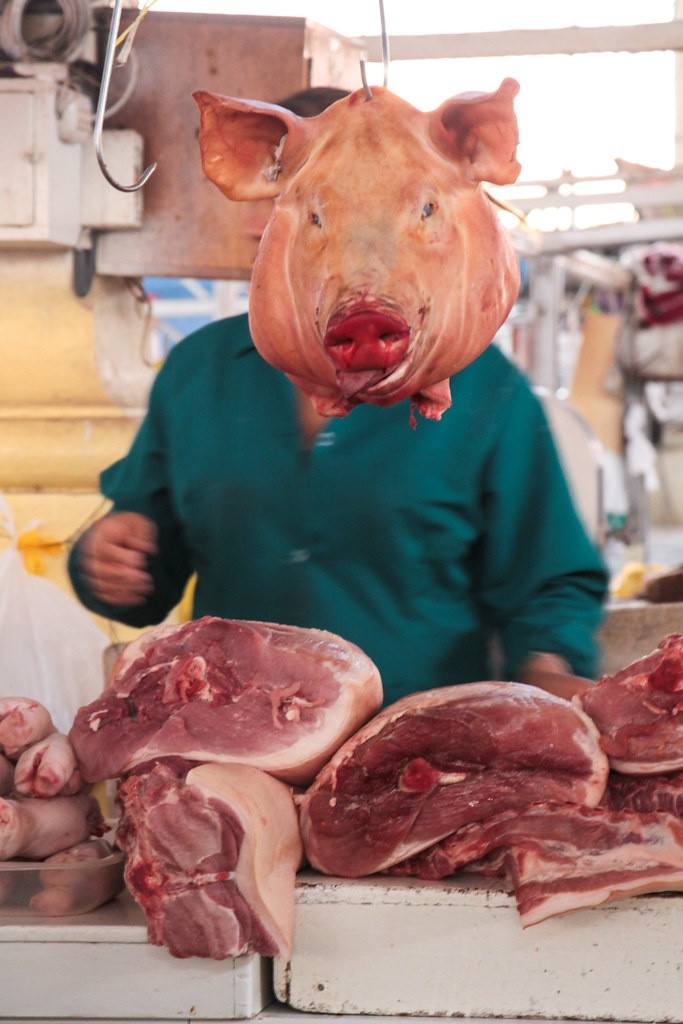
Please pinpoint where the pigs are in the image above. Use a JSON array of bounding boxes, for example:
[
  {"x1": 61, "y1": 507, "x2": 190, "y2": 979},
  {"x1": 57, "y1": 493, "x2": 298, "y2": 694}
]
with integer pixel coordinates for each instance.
[{"x1": 191, "y1": 78, "x2": 521, "y2": 419}]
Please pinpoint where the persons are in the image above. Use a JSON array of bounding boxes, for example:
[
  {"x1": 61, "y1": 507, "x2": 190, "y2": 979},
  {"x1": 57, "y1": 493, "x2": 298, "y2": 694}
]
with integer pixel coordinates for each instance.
[{"x1": 67, "y1": 90, "x2": 609, "y2": 703}]
